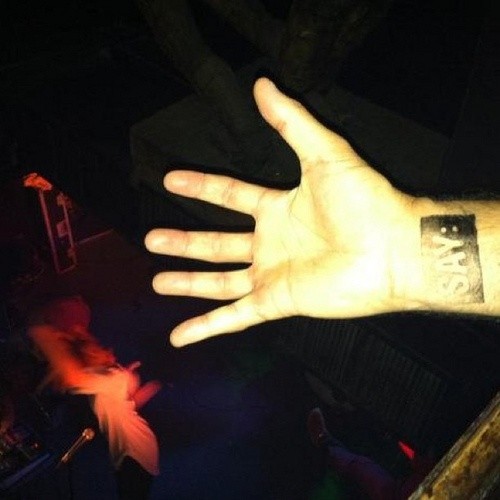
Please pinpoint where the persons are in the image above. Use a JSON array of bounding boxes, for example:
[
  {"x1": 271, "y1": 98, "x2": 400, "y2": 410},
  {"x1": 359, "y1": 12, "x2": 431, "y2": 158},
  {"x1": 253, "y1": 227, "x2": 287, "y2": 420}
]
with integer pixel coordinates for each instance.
[
  {"x1": 141, "y1": 79, "x2": 500, "y2": 349},
  {"x1": 16, "y1": 271, "x2": 178, "y2": 473},
  {"x1": 306, "y1": 402, "x2": 401, "y2": 500}
]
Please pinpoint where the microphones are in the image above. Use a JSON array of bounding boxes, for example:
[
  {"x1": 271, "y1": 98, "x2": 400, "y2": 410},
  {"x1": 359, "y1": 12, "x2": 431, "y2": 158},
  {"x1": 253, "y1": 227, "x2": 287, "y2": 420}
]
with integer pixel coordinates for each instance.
[{"x1": 57, "y1": 427, "x2": 95, "y2": 466}]
